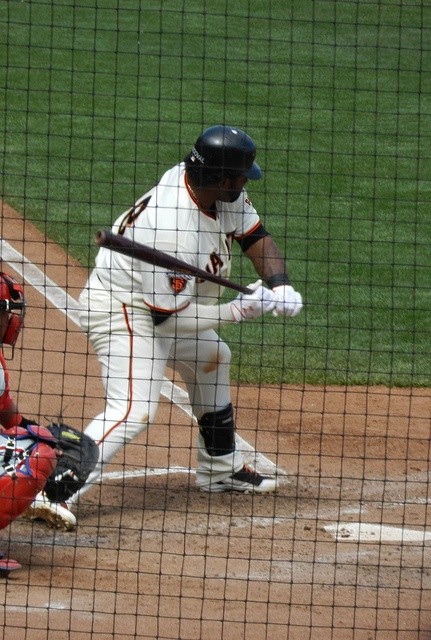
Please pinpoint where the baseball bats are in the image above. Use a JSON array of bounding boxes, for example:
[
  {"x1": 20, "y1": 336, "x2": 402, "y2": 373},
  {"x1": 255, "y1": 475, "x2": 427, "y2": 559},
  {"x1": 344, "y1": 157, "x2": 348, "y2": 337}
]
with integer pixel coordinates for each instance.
[{"x1": 96, "y1": 230, "x2": 253, "y2": 295}]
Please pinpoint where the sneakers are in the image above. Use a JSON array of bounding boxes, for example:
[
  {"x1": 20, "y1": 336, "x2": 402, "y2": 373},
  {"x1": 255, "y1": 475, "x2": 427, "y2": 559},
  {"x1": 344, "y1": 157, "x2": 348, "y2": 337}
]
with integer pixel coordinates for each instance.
[
  {"x1": 19, "y1": 492, "x2": 76, "y2": 532},
  {"x1": 196, "y1": 462, "x2": 280, "y2": 493}
]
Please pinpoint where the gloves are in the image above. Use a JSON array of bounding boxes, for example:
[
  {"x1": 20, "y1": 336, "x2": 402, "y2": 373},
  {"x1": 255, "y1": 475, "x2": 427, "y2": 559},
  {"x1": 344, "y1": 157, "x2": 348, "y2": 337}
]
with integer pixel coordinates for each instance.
[
  {"x1": 227, "y1": 278, "x2": 277, "y2": 323},
  {"x1": 271, "y1": 285, "x2": 304, "y2": 318}
]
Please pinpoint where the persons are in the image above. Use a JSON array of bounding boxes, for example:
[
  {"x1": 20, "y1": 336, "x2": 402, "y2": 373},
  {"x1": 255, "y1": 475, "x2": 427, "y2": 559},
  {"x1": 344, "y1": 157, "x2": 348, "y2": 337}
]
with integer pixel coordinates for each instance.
[
  {"x1": 0, "y1": 269, "x2": 65, "y2": 574},
  {"x1": 21, "y1": 126, "x2": 303, "y2": 531}
]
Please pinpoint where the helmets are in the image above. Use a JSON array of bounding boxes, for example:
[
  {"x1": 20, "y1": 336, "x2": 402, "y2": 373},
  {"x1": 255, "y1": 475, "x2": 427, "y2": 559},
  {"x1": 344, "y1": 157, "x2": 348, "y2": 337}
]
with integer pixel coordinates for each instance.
[
  {"x1": 185, "y1": 125, "x2": 262, "y2": 181},
  {"x1": 0, "y1": 271, "x2": 26, "y2": 346}
]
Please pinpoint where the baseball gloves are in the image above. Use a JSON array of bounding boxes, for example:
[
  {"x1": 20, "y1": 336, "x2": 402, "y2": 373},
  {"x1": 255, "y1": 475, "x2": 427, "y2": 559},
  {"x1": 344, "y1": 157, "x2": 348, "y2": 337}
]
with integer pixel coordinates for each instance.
[{"x1": 45, "y1": 423, "x2": 99, "y2": 503}]
